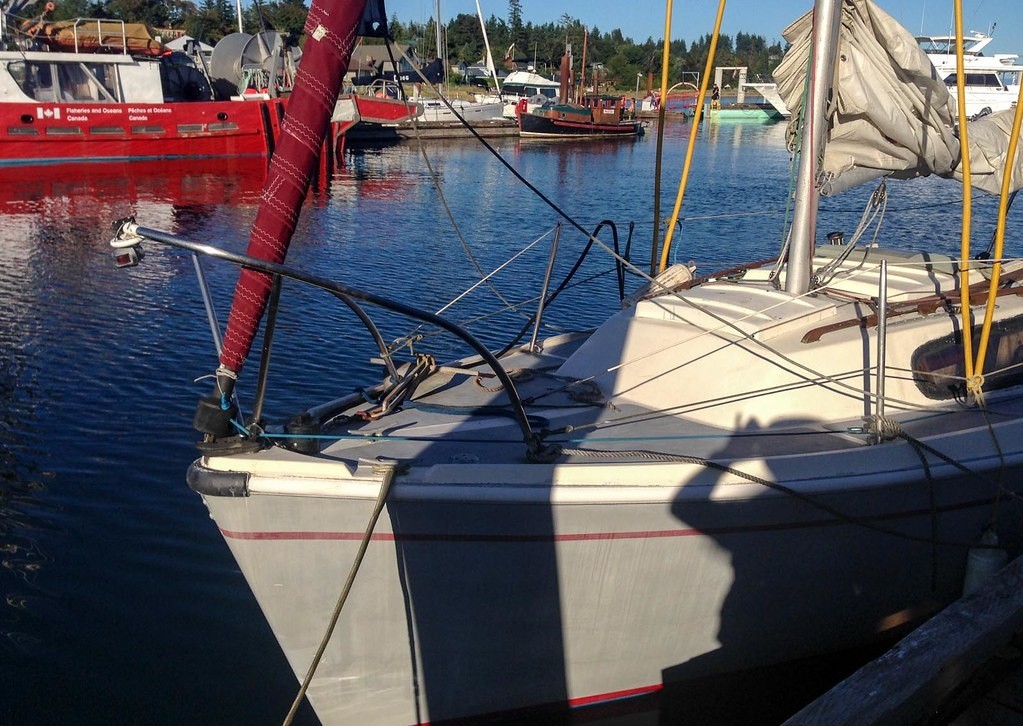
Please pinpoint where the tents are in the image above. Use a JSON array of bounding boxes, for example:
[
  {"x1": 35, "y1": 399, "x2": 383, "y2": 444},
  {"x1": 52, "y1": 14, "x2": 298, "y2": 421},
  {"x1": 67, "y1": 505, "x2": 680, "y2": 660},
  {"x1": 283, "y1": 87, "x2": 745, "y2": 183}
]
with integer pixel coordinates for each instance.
[{"x1": 164, "y1": 35, "x2": 213, "y2": 56}]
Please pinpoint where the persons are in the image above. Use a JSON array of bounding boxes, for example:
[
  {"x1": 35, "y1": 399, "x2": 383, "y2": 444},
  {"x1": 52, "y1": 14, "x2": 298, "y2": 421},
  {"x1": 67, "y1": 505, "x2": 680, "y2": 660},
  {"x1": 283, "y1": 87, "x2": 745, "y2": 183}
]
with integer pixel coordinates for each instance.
[
  {"x1": 711, "y1": 83, "x2": 720, "y2": 104},
  {"x1": 627, "y1": 98, "x2": 636, "y2": 120}
]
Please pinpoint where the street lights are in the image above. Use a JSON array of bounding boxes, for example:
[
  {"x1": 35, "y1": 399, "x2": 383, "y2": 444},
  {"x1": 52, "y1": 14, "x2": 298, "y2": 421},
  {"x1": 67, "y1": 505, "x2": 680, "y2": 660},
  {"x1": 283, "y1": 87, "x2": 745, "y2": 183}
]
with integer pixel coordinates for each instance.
[{"x1": 397, "y1": 0, "x2": 506, "y2": 124}]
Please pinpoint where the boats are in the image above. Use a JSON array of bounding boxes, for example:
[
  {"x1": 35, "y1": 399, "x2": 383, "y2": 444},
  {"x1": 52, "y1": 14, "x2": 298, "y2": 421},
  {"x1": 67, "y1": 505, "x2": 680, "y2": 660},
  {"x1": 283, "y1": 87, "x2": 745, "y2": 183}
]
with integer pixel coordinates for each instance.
[
  {"x1": 0, "y1": 0, "x2": 427, "y2": 167},
  {"x1": 513, "y1": 26, "x2": 647, "y2": 139},
  {"x1": 108, "y1": 0, "x2": 1023, "y2": 726},
  {"x1": 909, "y1": 19, "x2": 1023, "y2": 126},
  {"x1": 473, "y1": 39, "x2": 786, "y2": 121}
]
